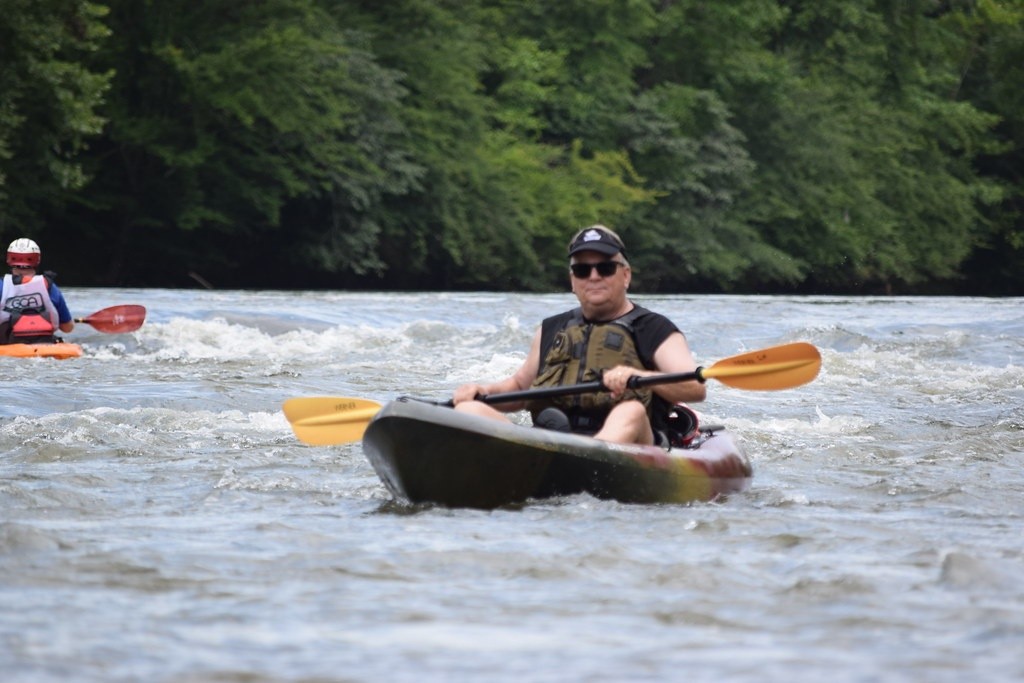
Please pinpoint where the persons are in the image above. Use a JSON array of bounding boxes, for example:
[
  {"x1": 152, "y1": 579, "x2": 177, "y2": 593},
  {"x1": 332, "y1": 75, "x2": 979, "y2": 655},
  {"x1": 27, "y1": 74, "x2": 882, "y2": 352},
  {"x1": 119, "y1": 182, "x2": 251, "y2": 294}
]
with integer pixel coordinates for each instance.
[
  {"x1": 0, "y1": 238, "x2": 74, "y2": 343},
  {"x1": 451, "y1": 223, "x2": 706, "y2": 443}
]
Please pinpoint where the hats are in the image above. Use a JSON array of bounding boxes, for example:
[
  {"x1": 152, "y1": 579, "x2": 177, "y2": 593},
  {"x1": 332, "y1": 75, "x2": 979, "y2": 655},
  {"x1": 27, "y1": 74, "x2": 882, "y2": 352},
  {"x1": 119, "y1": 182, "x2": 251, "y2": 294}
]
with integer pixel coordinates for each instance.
[{"x1": 567, "y1": 224, "x2": 630, "y2": 258}]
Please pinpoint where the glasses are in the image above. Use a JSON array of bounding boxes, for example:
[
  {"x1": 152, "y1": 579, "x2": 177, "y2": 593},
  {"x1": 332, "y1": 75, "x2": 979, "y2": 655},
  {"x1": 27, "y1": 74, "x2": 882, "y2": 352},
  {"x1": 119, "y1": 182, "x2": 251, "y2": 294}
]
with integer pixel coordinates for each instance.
[{"x1": 569, "y1": 261, "x2": 624, "y2": 278}]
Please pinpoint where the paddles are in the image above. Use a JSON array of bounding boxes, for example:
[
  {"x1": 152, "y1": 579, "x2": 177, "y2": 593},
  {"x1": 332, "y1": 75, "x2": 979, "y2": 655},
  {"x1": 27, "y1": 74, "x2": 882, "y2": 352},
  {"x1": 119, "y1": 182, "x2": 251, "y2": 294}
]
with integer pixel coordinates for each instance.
[
  {"x1": 52, "y1": 307, "x2": 147, "y2": 335},
  {"x1": 278, "y1": 344, "x2": 821, "y2": 448}
]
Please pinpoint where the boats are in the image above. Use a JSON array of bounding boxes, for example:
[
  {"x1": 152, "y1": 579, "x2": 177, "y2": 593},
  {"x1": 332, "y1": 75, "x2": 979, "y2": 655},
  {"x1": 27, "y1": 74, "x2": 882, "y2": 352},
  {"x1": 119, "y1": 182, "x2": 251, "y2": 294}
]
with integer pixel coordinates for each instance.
[
  {"x1": 1, "y1": 336, "x2": 87, "y2": 360},
  {"x1": 360, "y1": 397, "x2": 753, "y2": 511}
]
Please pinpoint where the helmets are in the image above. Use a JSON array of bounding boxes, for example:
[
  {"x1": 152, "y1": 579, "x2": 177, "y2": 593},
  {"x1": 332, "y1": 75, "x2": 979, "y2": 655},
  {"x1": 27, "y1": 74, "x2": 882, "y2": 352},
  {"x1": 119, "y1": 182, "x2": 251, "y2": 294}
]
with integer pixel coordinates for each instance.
[{"x1": 6, "y1": 237, "x2": 41, "y2": 266}]
